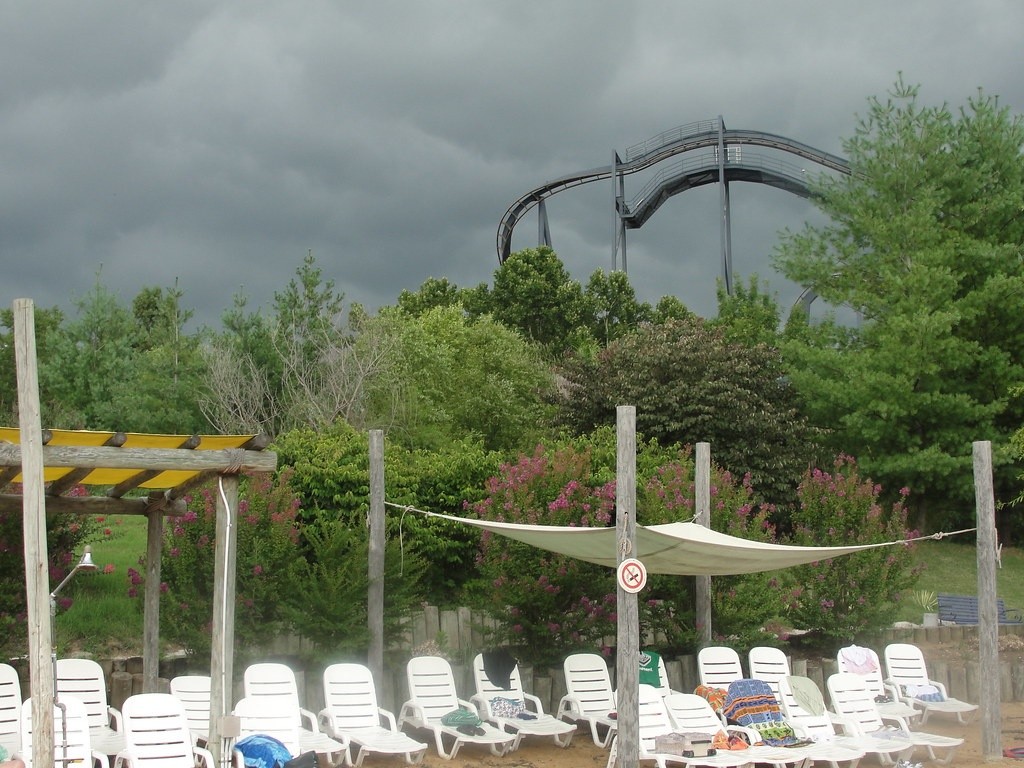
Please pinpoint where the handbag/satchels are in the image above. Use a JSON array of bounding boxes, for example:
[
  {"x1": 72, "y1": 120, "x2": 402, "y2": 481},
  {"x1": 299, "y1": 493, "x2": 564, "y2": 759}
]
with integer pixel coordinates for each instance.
[{"x1": 491, "y1": 696, "x2": 525, "y2": 718}]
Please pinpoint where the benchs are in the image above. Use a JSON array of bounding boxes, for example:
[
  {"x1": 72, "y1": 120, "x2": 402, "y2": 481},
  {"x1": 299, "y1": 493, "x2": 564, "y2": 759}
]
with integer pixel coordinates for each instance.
[{"x1": 936, "y1": 595, "x2": 1022, "y2": 623}]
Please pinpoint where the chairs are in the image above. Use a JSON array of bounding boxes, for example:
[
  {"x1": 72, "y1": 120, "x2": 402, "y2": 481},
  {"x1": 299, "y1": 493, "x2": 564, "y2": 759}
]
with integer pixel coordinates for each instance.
[
  {"x1": 0, "y1": 659, "x2": 428, "y2": 768},
  {"x1": 607, "y1": 643, "x2": 979, "y2": 768},
  {"x1": 468, "y1": 653, "x2": 577, "y2": 751},
  {"x1": 556, "y1": 654, "x2": 617, "y2": 747},
  {"x1": 397, "y1": 657, "x2": 516, "y2": 761}
]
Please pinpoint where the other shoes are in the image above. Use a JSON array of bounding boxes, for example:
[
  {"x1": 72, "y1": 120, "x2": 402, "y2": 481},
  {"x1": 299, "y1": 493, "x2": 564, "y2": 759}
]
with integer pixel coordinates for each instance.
[{"x1": 893, "y1": 759, "x2": 925, "y2": 768}]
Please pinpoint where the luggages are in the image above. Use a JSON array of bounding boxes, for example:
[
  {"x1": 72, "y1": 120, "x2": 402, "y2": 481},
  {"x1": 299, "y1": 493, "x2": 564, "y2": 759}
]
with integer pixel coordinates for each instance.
[{"x1": 655, "y1": 732, "x2": 717, "y2": 757}]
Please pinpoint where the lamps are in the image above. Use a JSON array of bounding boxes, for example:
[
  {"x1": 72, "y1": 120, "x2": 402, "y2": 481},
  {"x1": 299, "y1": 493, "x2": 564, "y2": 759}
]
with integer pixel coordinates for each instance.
[{"x1": 46, "y1": 545, "x2": 99, "y2": 604}]
